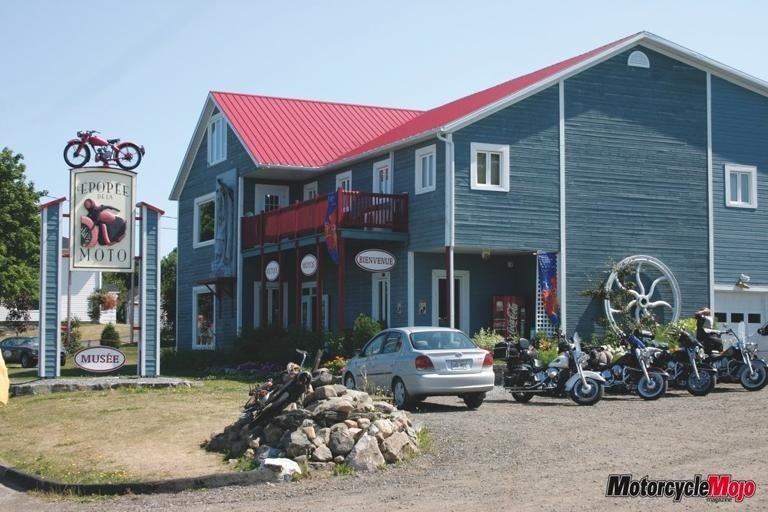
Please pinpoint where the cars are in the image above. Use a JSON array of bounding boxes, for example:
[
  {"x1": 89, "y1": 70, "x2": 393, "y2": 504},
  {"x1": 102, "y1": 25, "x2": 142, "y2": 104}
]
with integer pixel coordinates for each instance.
[
  {"x1": 342, "y1": 326, "x2": 496, "y2": 411},
  {"x1": 1, "y1": 336, "x2": 67, "y2": 368}
]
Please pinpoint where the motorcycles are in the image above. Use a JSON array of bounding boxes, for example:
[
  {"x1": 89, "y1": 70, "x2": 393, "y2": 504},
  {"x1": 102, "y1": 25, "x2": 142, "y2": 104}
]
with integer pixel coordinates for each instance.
[
  {"x1": 503, "y1": 309, "x2": 768, "y2": 404},
  {"x1": 63, "y1": 128, "x2": 146, "y2": 172},
  {"x1": 243, "y1": 347, "x2": 320, "y2": 429}
]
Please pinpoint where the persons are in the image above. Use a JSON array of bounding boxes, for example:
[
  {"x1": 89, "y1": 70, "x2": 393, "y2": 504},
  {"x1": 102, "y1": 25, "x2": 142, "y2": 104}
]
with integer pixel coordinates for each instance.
[{"x1": 695, "y1": 307, "x2": 724, "y2": 353}]
